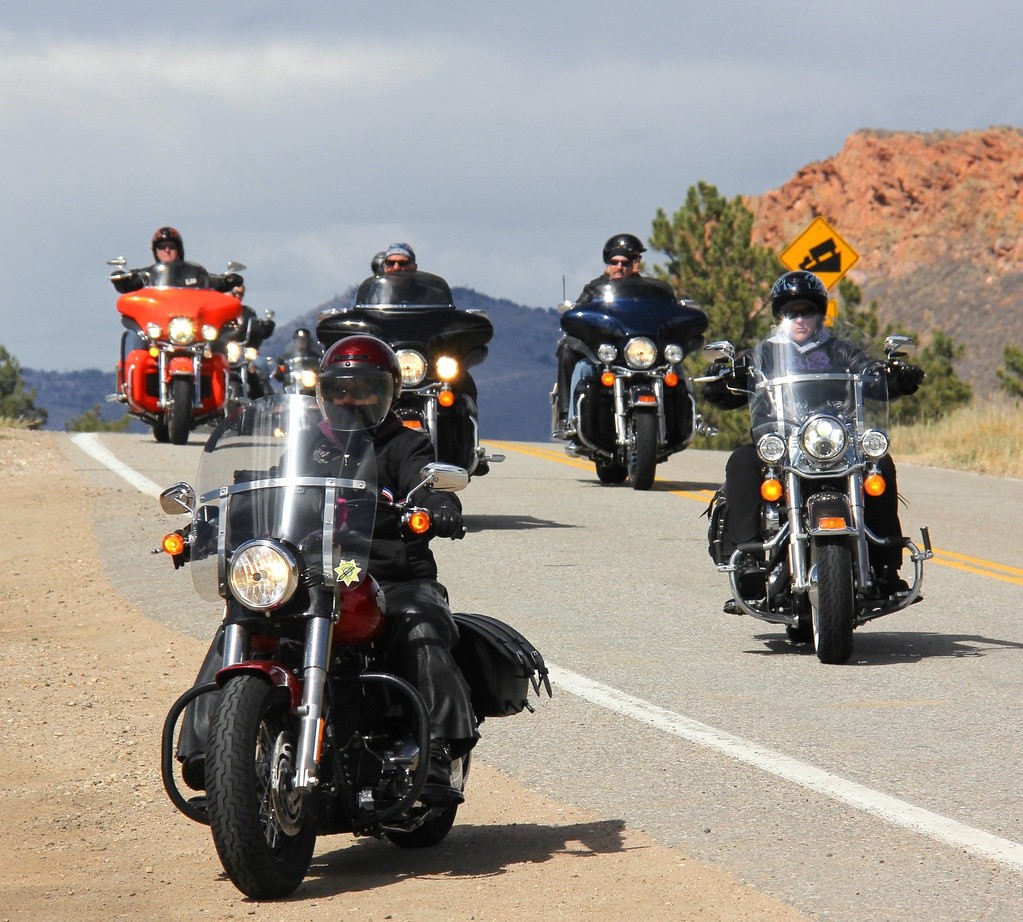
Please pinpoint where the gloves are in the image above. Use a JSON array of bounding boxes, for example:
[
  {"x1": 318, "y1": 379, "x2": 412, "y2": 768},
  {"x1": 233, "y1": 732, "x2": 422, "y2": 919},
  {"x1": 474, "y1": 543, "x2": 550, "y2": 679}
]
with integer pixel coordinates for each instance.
[
  {"x1": 427, "y1": 501, "x2": 463, "y2": 540},
  {"x1": 706, "y1": 364, "x2": 726, "y2": 377},
  {"x1": 225, "y1": 271, "x2": 243, "y2": 291},
  {"x1": 171, "y1": 529, "x2": 208, "y2": 570},
  {"x1": 896, "y1": 364, "x2": 922, "y2": 389},
  {"x1": 110, "y1": 270, "x2": 128, "y2": 285}
]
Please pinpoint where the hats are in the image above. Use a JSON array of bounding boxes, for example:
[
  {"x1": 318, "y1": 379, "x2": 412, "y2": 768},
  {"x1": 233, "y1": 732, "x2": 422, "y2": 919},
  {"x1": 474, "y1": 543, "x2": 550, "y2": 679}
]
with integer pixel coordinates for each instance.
[{"x1": 387, "y1": 243, "x2": 415, "y2": 264}]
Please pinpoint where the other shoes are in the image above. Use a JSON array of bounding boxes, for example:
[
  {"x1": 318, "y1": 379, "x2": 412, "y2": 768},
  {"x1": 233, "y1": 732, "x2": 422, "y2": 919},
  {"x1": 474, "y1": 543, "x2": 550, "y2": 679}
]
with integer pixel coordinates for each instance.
[
  {"x1": 413, "y1": 740, "x2": 451, "y2": 784},
  {"x1": 183, "y1": 754, "x2": 206, "y2": 791},
  {"x1": 879, "y1": 569, "x2": 909, "y2": 598},
  {"x1": 735, "y1": 552, "x2": 764, "y2": 598}
]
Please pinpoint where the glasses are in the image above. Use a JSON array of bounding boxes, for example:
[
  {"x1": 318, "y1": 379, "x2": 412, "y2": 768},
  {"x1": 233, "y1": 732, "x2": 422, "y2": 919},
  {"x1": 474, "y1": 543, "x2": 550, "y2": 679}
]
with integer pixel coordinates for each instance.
[
  {"x1": 156, "y1": 245, "x2": 179, "y2": 250},
  {"x1": 608, "y1": 259, "x2": 630, "y2": 266},
  {"x1": 784, "y1": 306, "x2": 817, "y2": 319},
  {"x1": 233, "y1": 290, "x2": 243, "y2": 297},
  {"x1": 631, "y1": 255, "x2": 642, "y2": 260},
  {"x1": 384, "y1": 260, "x2": 411, "y2": 267},
  {"x1": 327, "y1": 384, "x2": 379, "y2": 399}
]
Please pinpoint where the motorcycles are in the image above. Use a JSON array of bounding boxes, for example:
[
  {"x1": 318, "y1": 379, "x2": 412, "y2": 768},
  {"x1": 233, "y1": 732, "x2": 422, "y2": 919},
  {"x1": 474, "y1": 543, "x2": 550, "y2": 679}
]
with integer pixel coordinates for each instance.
[
  {"x1": 105, "y1": 253, "x2": 249, "y2": 446},
  {"x1": 232, "y1": 269, "x2": 507, "y2": 492},
  {"x1": 691, "y1": 313, "x2": 935, "y2": 667},
  {"x1": 548, "y1": 279, "x2": 709, "y2": 493},
  {"x1": 149, "y1": 384, "x2": 480, "y2": 904}
]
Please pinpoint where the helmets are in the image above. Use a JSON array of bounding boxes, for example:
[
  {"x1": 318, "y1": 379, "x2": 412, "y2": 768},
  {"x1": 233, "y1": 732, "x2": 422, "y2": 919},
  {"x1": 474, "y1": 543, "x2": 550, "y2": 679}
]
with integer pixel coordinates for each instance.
[
  {"x1": 770, "y1": 270, "x2": 828, "y2": 317},
  {"x1": 315, "y1": 335, "x2": 402, "y2": 432},
  {"x1": 372, "y1": 252, "x2": 387, "y2": 276},
  {"x1": 152, "y1": 227, "x2": 184, "y2": 262},
  {"x1": 603, "y1": 233, "x2": 647, "y2": 263},
  {"x1": 293, "y1": 327, "x2": 313, "y2": 351}
]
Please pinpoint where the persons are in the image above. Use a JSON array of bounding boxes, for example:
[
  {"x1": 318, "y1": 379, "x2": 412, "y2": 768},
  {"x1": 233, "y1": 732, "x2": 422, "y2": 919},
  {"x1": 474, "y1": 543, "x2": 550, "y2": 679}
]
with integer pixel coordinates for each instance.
[
  {"x1": 701, "y1": 270, "x2": 923, "y2": 615},
  {"x1": 105, "y1": 226, "x2": 244, "y2": 406},
  {"x1": 218, "y1": 284, "x2": 321, "y2": 402},
  {"x1": 553, "y1": 234, "x2": 685, "y2": 431},
  {"x1": 317, "y1": 245, "x2": 488, "y2": 319},
  {"x1": 172, "y1": 335, "x2": 478, "y2": 807}
]
